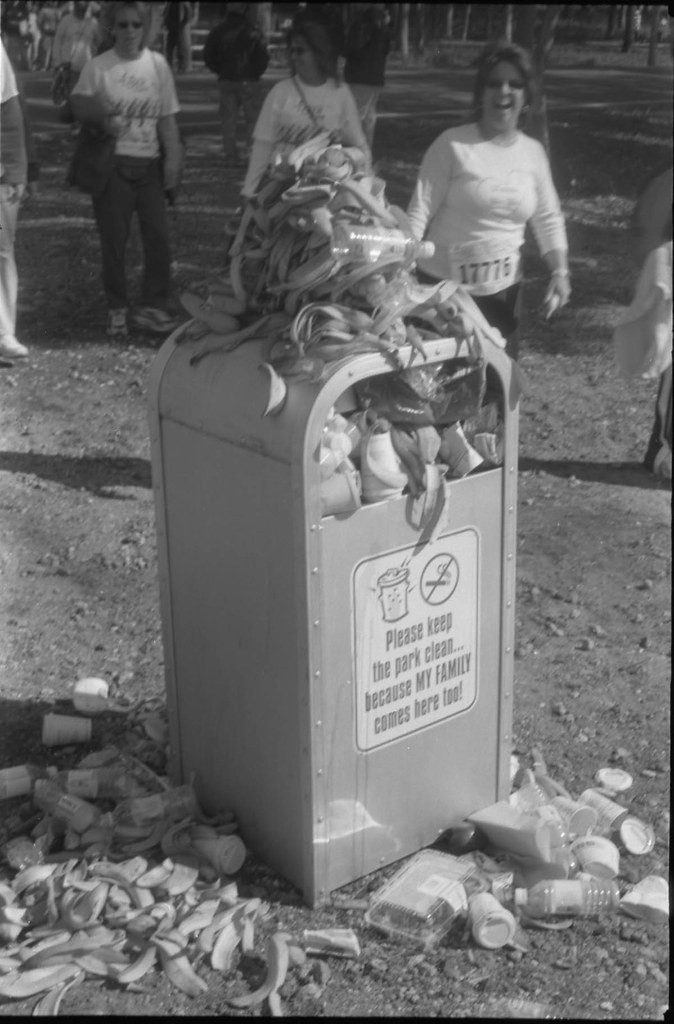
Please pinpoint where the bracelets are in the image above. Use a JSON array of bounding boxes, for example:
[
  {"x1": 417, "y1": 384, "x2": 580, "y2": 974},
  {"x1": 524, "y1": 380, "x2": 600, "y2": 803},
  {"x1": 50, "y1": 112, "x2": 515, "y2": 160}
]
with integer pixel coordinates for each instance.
[{"x1": 550, "y1": 268, "x2": 571, "y2": 278}]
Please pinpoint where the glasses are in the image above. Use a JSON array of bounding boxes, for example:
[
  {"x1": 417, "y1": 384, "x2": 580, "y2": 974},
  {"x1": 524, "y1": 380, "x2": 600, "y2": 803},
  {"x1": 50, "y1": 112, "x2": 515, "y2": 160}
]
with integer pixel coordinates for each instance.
[
  {"x1": 117, "y1": 21, "x2": 143, "y2": 29},
  {"x1": 486, "y1": 78, "x2": 525, "y2": 90},
  {"x1": 289, "y1": 46, "x2": 313, "y2": 55}
]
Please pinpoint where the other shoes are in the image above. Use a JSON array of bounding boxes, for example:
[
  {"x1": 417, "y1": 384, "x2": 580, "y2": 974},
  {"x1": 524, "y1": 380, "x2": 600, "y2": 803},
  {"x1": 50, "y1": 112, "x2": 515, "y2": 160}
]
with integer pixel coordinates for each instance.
[{"x1": 0, "y1": 335, "x2": 28, "y2": 364}]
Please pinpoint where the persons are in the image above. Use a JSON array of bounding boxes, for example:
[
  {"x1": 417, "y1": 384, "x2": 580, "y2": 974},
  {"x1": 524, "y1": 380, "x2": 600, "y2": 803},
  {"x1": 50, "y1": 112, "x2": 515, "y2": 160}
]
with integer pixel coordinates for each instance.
[
  {"x1": 345, "y1": 0, "x2": 392, "y2": 148},
  {"x1": 200, "y1": 0, "x2": 271, "y2": 157},
  {"x1": 406, "y1": 42, "x2": 572, "y2": 362},
  {"x1": 0, "y1": 36, "x2": 28, "y2": 366},
  {"x1": 69, "y1": 0, "x2": 183, "y2": 335},
  {"x1": 241, "y1": 10, "x2": 374, "y2": 198},
  {"x1": 614, "y1": 167, "x2": 674, "y2": 480},
  {"x1": 18, "y1": 0, "x2": 199, "y2": 74}
]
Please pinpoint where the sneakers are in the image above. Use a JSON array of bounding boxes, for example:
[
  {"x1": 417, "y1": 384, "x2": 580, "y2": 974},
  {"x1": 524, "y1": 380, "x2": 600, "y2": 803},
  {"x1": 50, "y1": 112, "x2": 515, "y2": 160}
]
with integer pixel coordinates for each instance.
[
  {"x1": 133, "y1": 305, "x2": 177, "y2": 332},
  {"x1": 104, "y1": 307, "x2": 128, "y2": 338}
]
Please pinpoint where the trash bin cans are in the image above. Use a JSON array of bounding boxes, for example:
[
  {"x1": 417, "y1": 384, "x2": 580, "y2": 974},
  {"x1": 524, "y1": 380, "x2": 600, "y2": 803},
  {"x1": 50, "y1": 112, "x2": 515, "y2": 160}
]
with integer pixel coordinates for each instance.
[{"x1": 149, "y1": 307, "x2": 519, "y2": 909}]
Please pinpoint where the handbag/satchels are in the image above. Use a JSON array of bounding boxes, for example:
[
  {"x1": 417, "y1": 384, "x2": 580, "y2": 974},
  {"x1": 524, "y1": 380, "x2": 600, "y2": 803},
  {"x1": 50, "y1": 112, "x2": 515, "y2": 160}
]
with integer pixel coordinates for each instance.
[
  {"x1": 52, "y1": 63, "x2": 73, "y2": 100},
  {"x1": 58, "y1": 140, "x2": 109, "y2": 195}
]
